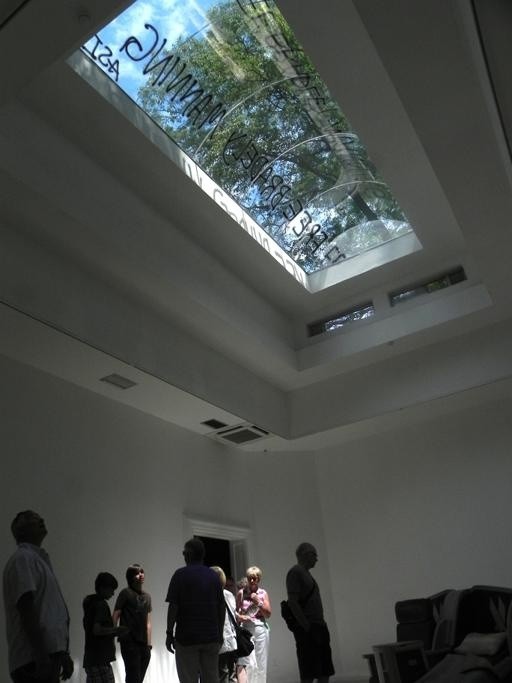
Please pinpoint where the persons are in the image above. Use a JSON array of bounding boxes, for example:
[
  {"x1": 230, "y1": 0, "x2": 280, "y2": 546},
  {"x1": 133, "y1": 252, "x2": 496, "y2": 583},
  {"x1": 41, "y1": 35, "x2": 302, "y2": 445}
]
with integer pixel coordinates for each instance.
[
  {"x1": 280, "y1": 543, "x2": 335, "y2": 683},
  {"x1": 82, "y1": 572, "x2": 130, "y2": 683},
  {"x1": 111, "y1": 564, "x2": 153, "y2": 683},
  {"x1": 3, "y1": 509, "x2": 74, "y2": 683},
  {"x1": 166, "y1": 539, "x2": 271, "y2": 683}
]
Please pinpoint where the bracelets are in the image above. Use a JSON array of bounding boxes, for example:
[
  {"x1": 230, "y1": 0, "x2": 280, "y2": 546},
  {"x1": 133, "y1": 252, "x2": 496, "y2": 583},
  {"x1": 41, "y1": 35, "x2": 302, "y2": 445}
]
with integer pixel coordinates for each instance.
[{"x1": 148, "y1": 645, "x2": 152, "y2": 649}]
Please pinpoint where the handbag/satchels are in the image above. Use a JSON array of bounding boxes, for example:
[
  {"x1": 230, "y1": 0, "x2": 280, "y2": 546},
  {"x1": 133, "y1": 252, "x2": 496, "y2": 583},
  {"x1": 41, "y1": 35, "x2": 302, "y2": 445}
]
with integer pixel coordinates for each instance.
[
  {"x1": 280, "y1": 598, "x2": 299, "y2": 632},
  {"x1": 235, "y1": 624, "x2": 254, "y2": 659}
]
